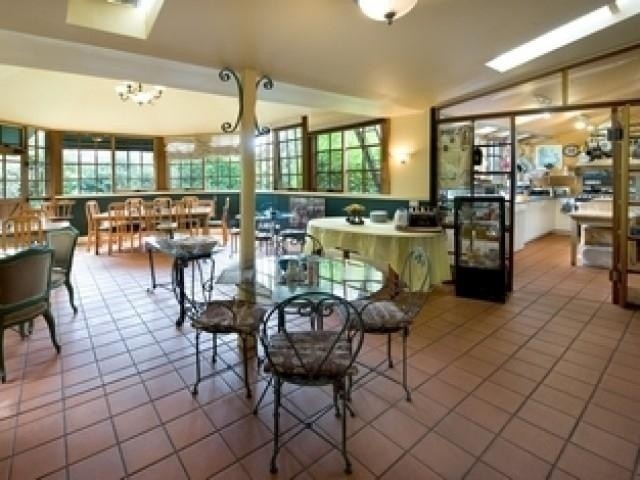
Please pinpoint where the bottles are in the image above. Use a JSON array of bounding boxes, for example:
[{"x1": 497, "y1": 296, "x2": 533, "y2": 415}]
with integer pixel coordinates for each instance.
[{"x1": 410, "y1": 207, "x2": 437, "y2": 227}]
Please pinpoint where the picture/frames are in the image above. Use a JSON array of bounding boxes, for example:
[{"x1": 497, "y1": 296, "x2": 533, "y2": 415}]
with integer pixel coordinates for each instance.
[
  {"x1": 535, "y1": 143, "x2": 563, "y2": 170},
  {"x1": 563, "y1": 143, "x2": 581, "y2": 157}
]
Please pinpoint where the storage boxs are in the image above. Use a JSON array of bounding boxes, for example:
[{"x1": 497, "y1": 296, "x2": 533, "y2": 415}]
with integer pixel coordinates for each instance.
[{"x1": 576, "y1": 224, "x2": 637, "y2": 266}]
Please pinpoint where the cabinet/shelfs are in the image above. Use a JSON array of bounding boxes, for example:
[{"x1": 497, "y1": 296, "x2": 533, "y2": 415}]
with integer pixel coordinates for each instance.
[
  {"x1": 572, "y1": 134, "x2": 640, "y2": 205},
  {"x1": 449, "y1": 195, "x2": 505, "y2": 304}
]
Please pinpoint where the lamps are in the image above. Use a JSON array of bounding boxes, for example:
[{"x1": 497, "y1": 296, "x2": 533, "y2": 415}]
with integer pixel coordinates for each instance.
[
  {"x1": 353, "y1": 0, "x2": 420, "y2": 27},
  {"x1": 396, "y1": 148, "x2": 414, "y2": 165},
  {"x1": 116, "y1": 81, "x2": 163, "y2": 107}
]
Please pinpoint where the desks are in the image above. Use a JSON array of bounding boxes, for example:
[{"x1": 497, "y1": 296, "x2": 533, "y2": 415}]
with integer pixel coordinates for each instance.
[
  {"x1": 302, "y1": 215, "x2": 453, "y2": 294},
  {"x1": 569, "y1": 209, "x2": 635, "y2": 266}
]
